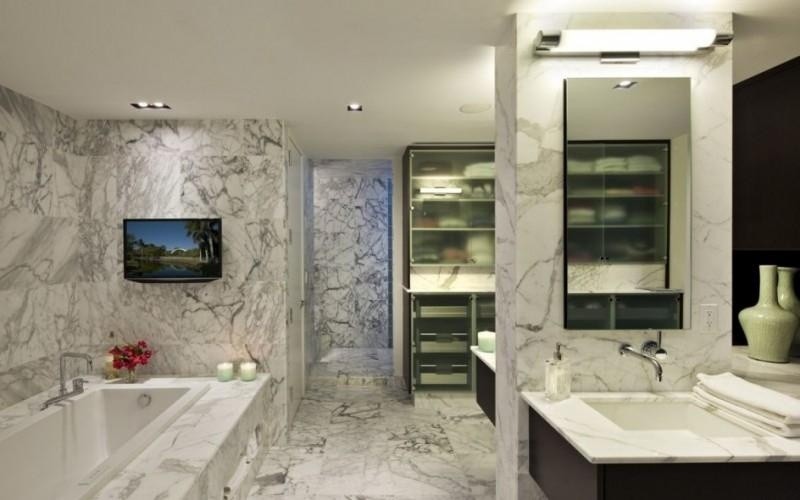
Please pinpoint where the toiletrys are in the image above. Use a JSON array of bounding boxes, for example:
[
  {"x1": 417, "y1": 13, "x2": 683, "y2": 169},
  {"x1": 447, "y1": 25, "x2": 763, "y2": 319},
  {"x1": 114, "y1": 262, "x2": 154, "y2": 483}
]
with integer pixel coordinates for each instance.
[
  {"x1": 545, "y1": 341, "x2": 572, "y2": 401},
  {"x1": 101, "y1": 331, "x2": 121, "y2": 381}
]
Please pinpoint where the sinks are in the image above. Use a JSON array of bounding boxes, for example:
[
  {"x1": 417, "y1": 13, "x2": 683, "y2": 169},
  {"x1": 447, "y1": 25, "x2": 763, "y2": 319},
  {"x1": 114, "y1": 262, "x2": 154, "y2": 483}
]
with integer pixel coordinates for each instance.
[
  {"x1": 0, "y1": 384, "x2": 212, "y2": 500},
  {"x1": 582, "y1": 395, "x2": 761, "y2": 432}
]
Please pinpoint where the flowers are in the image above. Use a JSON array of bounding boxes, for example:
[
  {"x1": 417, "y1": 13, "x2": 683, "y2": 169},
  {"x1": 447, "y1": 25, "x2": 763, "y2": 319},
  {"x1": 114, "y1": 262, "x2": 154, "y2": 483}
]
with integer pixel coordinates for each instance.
[{"x1": 109, "y1": 339, "x2": 153, "y2": 370}]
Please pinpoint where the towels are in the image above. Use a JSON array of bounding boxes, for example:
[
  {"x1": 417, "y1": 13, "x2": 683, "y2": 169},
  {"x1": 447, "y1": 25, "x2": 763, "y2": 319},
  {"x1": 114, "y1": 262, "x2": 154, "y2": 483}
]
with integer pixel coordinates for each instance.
[
  {"x1": 690, "y1": 371, "x2": 799, "y2": 442},
  {"x1": 438, "y1": 218, "x2": 467, "y2": 227},
  {"x1": 463, "y1": 160, "x2": 497, "y2": 176},
  {"x1": 568, "y1": 153, "x2": 661, "y2": 174},
  {"x1": 466, "y1": 238, "x2": 495, "y2": 263},
  {"x1": 569, "y1": 203, "x2": 625, "y2": 225}
]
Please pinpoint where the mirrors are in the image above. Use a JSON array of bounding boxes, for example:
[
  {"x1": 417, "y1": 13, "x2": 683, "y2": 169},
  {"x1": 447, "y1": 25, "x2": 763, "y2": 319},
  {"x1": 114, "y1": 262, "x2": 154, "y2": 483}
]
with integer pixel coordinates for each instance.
[{"x1": 563, "y1": 75, "x2": 693, "y2": 332}]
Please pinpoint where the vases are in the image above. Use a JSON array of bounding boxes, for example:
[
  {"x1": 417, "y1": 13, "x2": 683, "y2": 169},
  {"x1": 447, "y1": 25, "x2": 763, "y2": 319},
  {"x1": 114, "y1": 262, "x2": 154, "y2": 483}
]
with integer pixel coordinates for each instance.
[
  {"x1": 126, "y1": 362, "x2": 137, "y2": 384},
  {"x1": 776, "y1": 264, "x2": 800, "y2": 360},
  {"x1": 740, "y1": 262, "x2": 799, "y2": 365}
]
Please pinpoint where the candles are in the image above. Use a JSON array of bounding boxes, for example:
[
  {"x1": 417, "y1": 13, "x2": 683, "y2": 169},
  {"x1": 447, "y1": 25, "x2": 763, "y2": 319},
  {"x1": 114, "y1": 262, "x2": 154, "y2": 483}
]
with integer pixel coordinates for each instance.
[
  {"x1": 216, "y1": 362, "x2": 235, "y2": 382},
  {"x1": 239, "y1": 361, "x2": 257, "y2": 381},
  {"x1": 475, "y1": 327, "x2": 496, "y2": 353}
]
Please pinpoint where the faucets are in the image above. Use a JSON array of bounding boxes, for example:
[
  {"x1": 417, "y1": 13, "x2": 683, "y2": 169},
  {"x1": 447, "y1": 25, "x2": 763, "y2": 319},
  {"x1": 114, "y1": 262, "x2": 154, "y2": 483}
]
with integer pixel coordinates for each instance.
[
  {"x1": 624, "y1": 346, "x2": 664, "y2": 382},
  {"x1": 58, "y1": 352, "x2": 93, "y2": 396}
]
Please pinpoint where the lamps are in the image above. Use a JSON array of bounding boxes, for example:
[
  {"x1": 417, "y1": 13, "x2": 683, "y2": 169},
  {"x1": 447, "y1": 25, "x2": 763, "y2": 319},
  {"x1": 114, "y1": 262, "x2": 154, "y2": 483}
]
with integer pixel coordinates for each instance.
[{"x1": 532, "y1": 30, "x2": 736, "y2": 65}]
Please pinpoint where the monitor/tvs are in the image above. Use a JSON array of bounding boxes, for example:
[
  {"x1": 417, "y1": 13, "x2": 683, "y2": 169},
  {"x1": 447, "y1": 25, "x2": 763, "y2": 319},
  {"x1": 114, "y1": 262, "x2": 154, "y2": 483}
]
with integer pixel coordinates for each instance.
[{"x1": 123, "y1": 218, "x2": 222, "y2": 283}]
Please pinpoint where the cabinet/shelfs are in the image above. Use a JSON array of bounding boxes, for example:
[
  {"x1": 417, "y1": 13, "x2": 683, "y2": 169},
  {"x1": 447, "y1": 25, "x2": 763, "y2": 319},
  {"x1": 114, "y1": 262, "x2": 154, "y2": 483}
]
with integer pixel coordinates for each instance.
[
  {"x1": 404, "y1": 290, "x2": 495, "y2": 396},
  {"x1": 565, "y1": 139, "x2": 668, "y2": 292},
  {"x1": 401, "y1": 142, "x2": 495, "y2": 268},
  {"x1": 564, "y1": 288, "x2": 683, "y2": 329}
]
